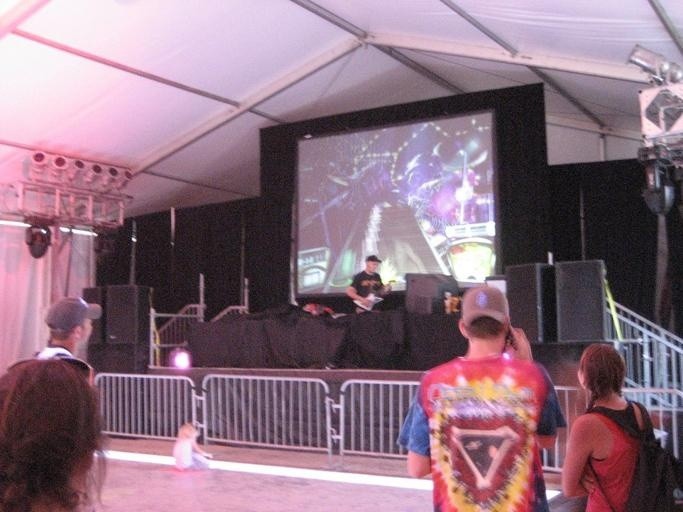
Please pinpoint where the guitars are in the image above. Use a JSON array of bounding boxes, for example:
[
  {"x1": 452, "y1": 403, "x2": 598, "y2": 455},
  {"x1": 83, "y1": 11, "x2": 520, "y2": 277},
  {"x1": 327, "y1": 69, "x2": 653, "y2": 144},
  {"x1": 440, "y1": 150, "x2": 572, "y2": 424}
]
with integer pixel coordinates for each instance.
[{"x1": 354, "y1": 279, "x2": 397, "y2": 310}]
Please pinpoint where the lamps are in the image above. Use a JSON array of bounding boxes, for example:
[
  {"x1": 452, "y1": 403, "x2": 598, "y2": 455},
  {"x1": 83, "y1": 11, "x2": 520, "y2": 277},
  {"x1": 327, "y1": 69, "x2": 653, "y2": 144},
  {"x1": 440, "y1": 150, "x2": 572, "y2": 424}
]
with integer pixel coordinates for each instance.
[
  {"x1": 624, "y1": 43, "x2": 683, "y2": 85},
  {"x1": 635, "y1": 143, "x2": 675, "y2": 219},
  {"x1": 28, "y1": 150, "x2": 132, "y2": 194},
  {"x1": 25, "y1": 222, "x2": 53, "y2": 260}
]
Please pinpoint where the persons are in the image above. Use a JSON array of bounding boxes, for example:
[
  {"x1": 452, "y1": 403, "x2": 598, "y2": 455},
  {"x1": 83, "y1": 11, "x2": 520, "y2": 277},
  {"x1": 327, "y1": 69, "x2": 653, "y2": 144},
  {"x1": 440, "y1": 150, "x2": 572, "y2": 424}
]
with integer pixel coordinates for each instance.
[
  {"x1": 31, "y1": 296, "x2": 102, "y2": 362},
  {"x1": 395, "y1": 283, "x2": 569, "y2": 511},
  {"x1": 344, "y1": 254, "x2": 392, "y2": 317},
  {"x1": 0, "y1": 356, "x2": 105, "y2": 511},
  {"x1": 560, "y1": 341, "x2": 657, "y2": 510},
  {"x1": 173, "y1": 422, "x2": 215, "y2": 471}
]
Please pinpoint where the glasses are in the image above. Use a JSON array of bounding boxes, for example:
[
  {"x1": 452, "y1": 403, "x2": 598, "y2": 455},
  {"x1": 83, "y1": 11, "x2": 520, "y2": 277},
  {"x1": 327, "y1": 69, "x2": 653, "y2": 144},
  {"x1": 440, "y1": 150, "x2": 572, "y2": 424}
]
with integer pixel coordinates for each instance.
[{"x1": 8, "y1": 355, "x2": 97, "y2": 389}]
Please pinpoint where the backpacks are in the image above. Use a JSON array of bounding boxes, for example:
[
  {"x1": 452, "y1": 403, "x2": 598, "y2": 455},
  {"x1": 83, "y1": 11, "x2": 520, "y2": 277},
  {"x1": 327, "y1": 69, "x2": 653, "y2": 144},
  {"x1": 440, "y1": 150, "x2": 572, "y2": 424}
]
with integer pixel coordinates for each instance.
[{"x1": 584, "y1": 400, "x2": 682, "y2": 512}]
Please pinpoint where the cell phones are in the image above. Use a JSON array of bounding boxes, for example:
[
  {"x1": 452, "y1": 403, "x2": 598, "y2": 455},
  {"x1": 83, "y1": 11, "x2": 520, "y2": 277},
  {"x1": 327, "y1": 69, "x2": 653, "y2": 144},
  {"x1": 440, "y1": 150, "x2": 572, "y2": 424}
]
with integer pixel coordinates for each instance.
[{"x1": 507, "y1": 324, "x2": 515, "y2": 347}]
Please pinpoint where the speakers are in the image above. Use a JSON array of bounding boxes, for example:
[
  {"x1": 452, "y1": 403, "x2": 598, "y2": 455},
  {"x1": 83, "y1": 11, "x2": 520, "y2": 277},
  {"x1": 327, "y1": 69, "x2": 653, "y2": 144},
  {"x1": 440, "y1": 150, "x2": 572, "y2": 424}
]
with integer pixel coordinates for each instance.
[
  {"x1": 81, "y1": 287, "x2": 104, "y2": 343},
  {"x1": 553, "y1": 260, "x2": 608, "y2": 340},
  {"x1": 504, "y1": 261, "x2": 552, "y2": 343},
  {"x1": 104, "y1": 285, "x2": 151, "y2": 344}
]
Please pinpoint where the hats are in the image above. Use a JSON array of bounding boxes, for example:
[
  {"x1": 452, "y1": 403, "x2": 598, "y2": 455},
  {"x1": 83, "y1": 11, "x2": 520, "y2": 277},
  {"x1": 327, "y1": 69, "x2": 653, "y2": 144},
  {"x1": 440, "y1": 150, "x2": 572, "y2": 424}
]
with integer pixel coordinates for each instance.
[
  {"x1": 367, "y1": 256, "x2": 381, "y2": 263},
  {"x1": 463, "y1": 287, "x2": 509, "y2": 326},
  {"x1": 45, "y1": 298, "x2": 103, "y2": 330}
]
